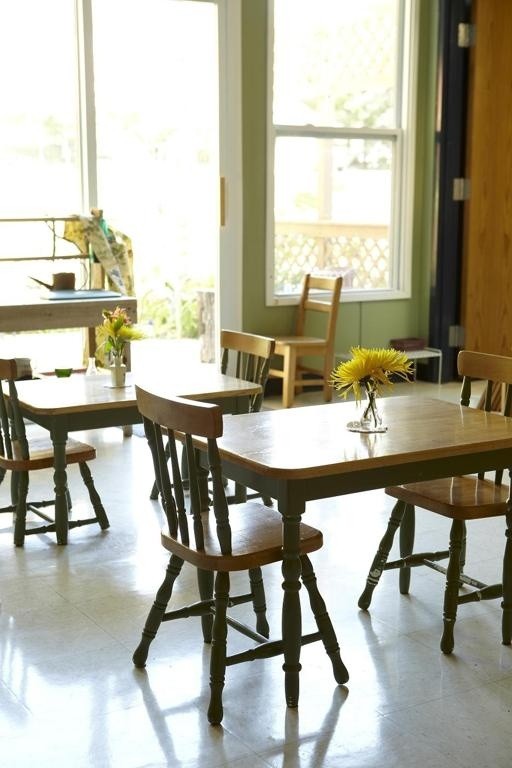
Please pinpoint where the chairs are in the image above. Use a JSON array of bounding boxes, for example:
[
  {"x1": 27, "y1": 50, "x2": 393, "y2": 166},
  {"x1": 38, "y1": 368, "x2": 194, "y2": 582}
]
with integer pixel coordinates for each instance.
[
  {"x1": 152, "y1": 327, "x2": 274, "y2": 505},
  {"x1": 129, "y1": 384, "x2": 348, "y2": 727},
  {"x1": 358, "y1": 350, "x2": 511, "y2": 654},
  {"x1": 253, "y1": 273, "x2": 344, "y2": 407},
  {"x1": 0, "y1": 360, "x2": 109, "y2": 546}
]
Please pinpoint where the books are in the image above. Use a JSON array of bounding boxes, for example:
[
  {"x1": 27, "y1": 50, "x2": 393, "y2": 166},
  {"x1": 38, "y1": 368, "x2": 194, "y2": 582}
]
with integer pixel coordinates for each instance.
[{"x1": 37, "y1": 289, "x2": 122, "y2": 300}]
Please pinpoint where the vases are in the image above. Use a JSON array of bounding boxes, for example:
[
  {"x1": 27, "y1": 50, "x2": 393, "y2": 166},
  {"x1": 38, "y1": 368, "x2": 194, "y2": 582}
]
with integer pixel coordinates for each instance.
[
  {"x1": 110, "y1": 357, "x2": 126, "y2": 387},
  {"x1": 347, "y1": 383, "x2": 389, "y2": 433}
]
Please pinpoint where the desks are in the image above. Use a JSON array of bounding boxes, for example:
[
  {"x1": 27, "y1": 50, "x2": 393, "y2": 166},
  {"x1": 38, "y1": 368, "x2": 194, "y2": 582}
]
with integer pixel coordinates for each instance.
[{"x1": 0, "y1": 290, "x2": 136, "y2": 437}]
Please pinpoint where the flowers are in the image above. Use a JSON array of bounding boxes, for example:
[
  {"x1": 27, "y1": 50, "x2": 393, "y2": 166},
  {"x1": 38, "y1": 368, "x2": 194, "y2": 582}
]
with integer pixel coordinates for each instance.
[
  {"x1": 94, "y1": 306, "x2": 147, "y2": 357},
  {"x1": 328, "y1": 346, "x2": 416, "y2": 427}
]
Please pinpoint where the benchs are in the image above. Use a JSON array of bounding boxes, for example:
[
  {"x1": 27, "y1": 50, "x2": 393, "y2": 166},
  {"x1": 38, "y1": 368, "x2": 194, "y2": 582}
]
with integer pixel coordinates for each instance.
[{"x1": 334, "y1": 347, "x2": 442, "y2": 383}]
